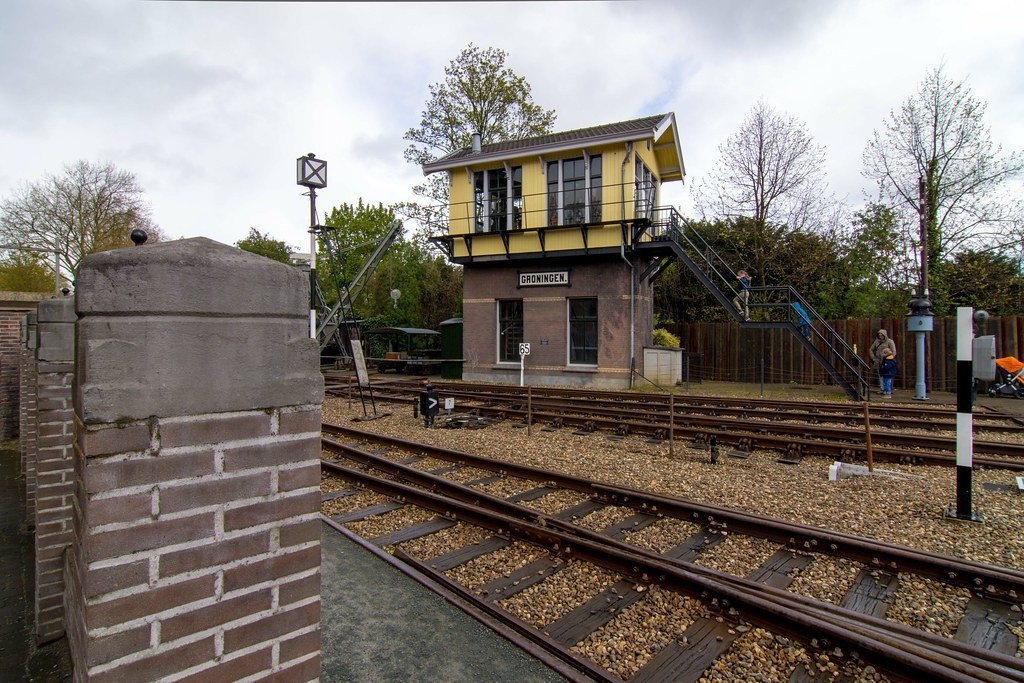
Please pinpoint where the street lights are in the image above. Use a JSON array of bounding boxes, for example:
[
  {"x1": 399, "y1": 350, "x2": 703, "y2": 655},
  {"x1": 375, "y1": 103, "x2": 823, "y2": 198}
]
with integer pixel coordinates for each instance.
[{"x1": 904, "y1": 288, "x2": 935, "y2": 402}]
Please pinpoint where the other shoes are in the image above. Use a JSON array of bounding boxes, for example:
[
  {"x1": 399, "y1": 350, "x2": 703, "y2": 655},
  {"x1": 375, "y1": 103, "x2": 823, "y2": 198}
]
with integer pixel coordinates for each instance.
[
  {"x1": 876, "y1": 389, "x2": 894, "y2": 399},
  {"x1": 805, "y1": 335, "x2": 811, "y2": 342},
  {"x1": 743, "y1": 317, "x2": 750, "y2": 321},
  {"x1": 738, "y1": 310, "x2": 744, "y2": 314}
]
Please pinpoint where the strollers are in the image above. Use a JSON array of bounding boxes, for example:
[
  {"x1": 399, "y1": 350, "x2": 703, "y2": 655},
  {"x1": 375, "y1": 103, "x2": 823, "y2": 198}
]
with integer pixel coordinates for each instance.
[{"x1": 989, "y1": 355, "x2": 1024, "y2": 400}]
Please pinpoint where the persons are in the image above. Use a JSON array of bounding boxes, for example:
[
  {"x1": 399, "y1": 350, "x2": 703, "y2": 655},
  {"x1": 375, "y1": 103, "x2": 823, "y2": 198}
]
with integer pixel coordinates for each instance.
[
  {"x1": 880, "y1": 348, "x2": 898, "y2": 400},
  {"x1": 793, "y1": 301, "x2": 812, "y2": 342},
  {"x1": 734, "y1": 270, "x2": 753, "y2": 322},
  {"x1": 870, "y1": 330, "x2": 898, "y2": 395}
]
